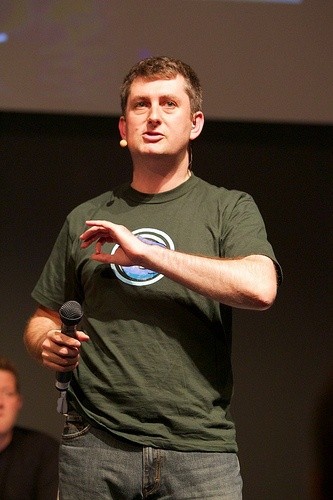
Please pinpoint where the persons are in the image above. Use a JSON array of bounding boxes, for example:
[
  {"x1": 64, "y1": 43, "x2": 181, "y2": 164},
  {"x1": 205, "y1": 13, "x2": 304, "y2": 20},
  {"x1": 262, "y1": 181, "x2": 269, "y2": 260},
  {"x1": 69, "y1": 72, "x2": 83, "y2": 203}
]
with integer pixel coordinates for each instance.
[
  {"x1": 23, "y1": 56, "x2": 284, "y2": 500},
  {"x1": 0, "y1": 363, "x2": 60, "y2": 500}
]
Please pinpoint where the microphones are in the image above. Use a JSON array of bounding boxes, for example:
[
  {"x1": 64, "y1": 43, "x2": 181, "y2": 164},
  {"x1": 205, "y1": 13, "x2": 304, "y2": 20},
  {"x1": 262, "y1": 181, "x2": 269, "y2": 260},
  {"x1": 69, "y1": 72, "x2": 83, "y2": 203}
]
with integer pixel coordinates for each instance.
[
  {"x1": 119, "y1": 140, "x2": 127, "y2": 147},
  {"x1": 56, "y1": 300, "x2": 84, "y2": 390}
]
